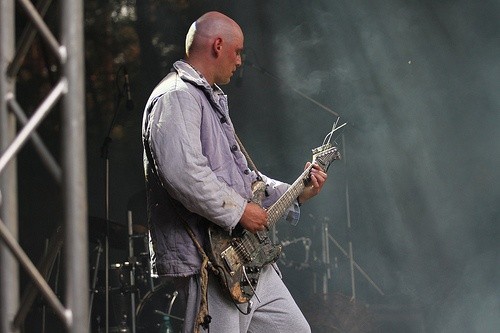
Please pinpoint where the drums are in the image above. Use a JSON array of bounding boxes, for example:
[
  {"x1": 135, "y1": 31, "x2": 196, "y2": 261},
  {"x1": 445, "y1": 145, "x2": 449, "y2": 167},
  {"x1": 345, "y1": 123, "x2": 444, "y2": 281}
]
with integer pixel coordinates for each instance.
[{"x1": 131, "y1": 281, "x2": 188, "y2": 333}]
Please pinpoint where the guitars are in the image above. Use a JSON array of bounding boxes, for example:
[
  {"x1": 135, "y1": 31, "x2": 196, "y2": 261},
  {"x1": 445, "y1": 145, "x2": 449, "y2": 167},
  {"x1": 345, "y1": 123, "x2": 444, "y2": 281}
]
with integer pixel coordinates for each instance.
[{"x1": 204, "y1": 139, "x2": 341, "y2": 306}]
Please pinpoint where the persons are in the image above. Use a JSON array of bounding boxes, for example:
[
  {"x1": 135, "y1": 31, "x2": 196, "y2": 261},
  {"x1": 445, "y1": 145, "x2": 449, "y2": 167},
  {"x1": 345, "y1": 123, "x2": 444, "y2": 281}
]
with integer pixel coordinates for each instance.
[{"x1": 136, "y1": 11, "x2": 328, "y2": 331}]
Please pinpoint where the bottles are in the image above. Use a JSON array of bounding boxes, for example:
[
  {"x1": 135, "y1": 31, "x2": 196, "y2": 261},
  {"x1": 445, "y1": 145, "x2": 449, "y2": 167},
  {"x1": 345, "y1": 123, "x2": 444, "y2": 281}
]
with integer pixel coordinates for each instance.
[{"x1": 161, "y1": 316, "x2": 172, "y2": 333}]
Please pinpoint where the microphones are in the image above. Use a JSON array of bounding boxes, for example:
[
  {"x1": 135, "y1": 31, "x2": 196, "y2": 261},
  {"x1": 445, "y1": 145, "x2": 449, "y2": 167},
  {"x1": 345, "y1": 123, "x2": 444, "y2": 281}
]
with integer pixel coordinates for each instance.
[{"x1": 123, "y1": 69, "x2": 135, "y2": 110}]
